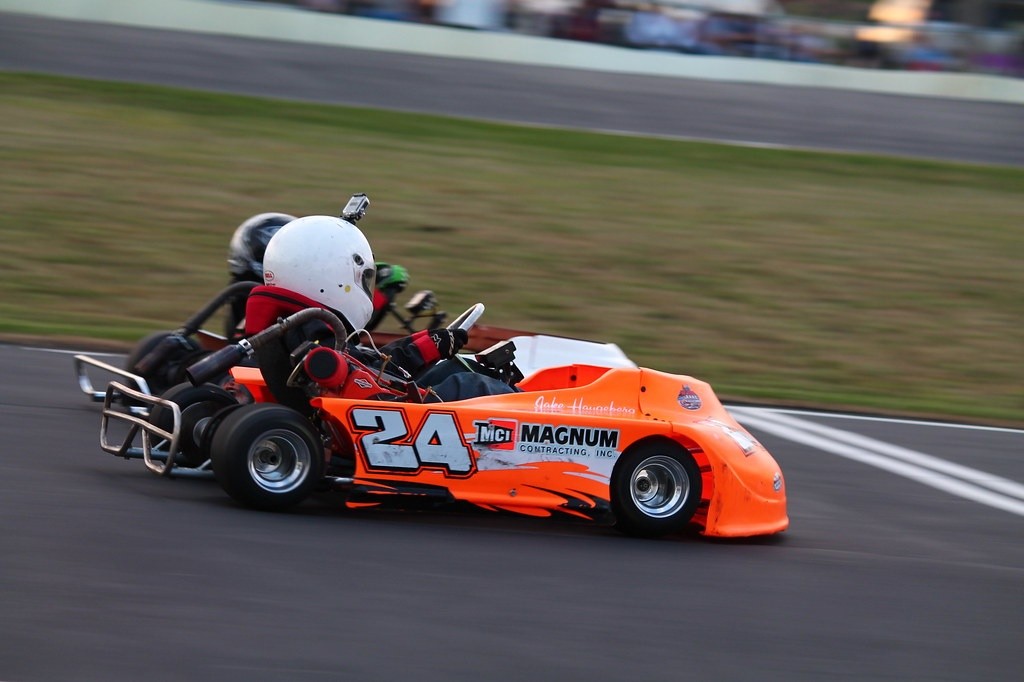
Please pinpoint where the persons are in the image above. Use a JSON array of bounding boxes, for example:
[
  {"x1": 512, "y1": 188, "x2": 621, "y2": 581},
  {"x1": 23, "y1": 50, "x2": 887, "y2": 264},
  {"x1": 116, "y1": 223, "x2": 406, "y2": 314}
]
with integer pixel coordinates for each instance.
[
  {"x1": 227, "y1": 213, "x2": 409, "y2": 344},
  {"x1": 263, "y1": 214, "x2": 524, "y2": 403}
]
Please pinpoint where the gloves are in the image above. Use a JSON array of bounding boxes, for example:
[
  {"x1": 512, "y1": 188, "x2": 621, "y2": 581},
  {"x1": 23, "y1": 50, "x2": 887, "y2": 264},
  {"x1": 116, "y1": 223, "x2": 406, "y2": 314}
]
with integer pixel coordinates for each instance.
[
  {"x1": 374, "y1": 262, "x2": 410, "y2": 294},
  {"x1": 429, "y1": 329, "x2": 468, "y2": 360}
]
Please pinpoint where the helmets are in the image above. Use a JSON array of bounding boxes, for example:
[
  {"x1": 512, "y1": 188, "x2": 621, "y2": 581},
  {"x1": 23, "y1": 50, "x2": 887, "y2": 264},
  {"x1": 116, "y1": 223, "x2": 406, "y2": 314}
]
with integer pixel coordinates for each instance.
[
  {"x1": 227, "y1": 212, "x2": 299, "y2": 278},
  {"x1": 262, "y1": 215, "x2": 377, "y2": 346}
]
